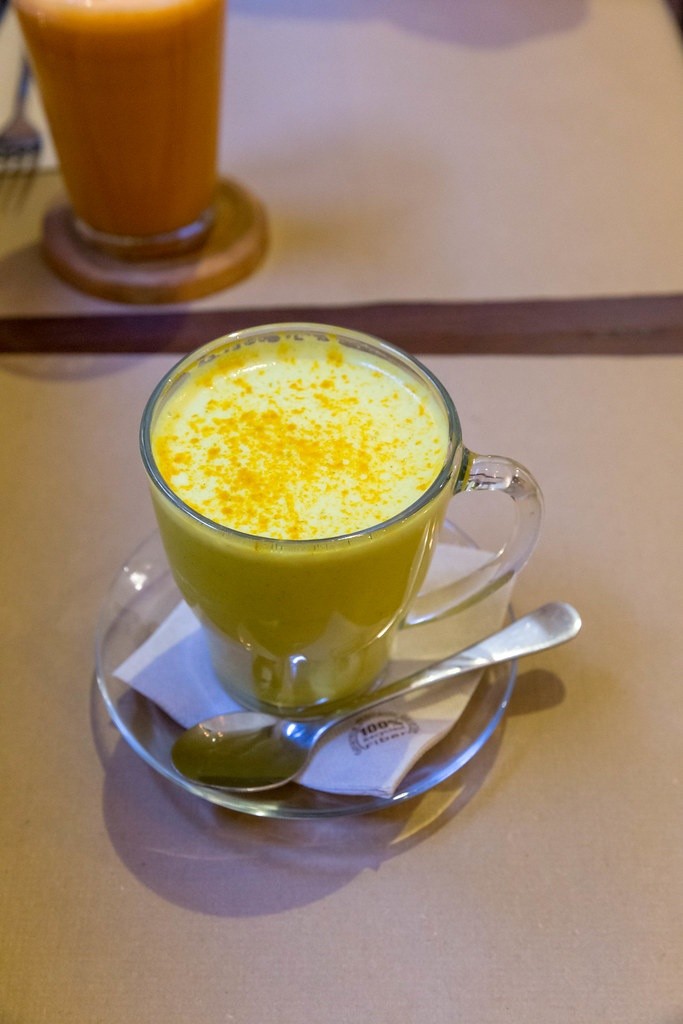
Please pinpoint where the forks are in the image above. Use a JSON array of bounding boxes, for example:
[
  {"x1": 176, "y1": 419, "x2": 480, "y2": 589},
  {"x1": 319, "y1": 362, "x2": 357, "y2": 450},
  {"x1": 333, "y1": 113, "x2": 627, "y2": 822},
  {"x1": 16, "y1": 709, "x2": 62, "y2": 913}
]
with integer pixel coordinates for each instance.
[{"x1": 0, "y1": 1, "x2": 43, "y2": 218}]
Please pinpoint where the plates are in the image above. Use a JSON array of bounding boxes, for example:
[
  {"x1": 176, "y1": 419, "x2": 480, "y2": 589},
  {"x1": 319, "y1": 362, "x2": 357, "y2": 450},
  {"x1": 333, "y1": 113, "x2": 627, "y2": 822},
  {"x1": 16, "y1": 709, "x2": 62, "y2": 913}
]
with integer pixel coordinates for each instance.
[{"x1": 95, "y1": 511, "x2": 520, "y2": 819}]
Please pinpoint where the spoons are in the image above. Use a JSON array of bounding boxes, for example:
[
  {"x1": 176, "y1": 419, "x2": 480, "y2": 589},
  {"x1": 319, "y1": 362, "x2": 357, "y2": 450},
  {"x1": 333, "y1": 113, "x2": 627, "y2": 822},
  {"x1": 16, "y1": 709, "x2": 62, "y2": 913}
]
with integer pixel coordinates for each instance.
[{"x1": 170, "y1": 599, "x2": 581, "y2": 792}]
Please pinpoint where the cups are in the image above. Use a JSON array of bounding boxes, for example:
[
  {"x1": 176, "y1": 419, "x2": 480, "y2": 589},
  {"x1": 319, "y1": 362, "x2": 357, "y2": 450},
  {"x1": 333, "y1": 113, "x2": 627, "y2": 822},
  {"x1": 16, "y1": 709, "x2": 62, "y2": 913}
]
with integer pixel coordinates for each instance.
[
  {"x1": 135, "y1": 319, "x2": 545, "y2": 724},
  {"x1": 10, "y1": 1, "x2": 230, "y2": 260}
]
